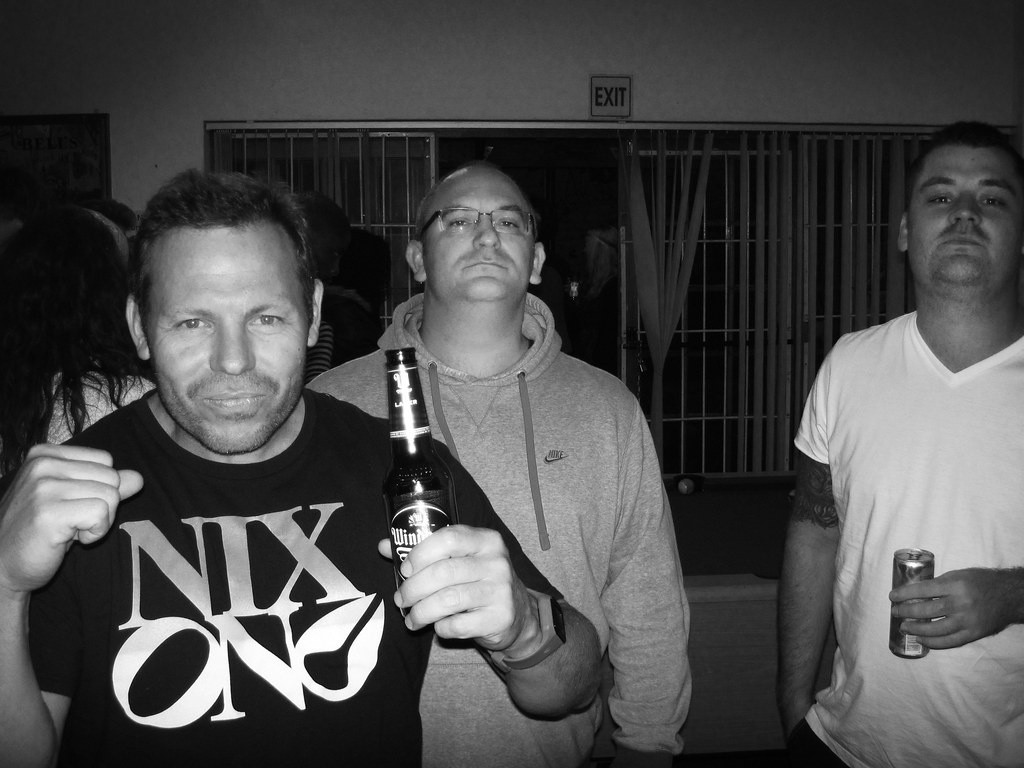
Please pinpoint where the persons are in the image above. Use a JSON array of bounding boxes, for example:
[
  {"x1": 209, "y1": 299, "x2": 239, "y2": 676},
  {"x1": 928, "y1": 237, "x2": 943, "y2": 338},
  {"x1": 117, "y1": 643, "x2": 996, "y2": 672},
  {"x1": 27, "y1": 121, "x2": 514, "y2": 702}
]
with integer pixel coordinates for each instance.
[
  {"x1": 778, "y1": 120, "x2": 1024, "y2": 767},
  {"x1": 0, "y1": 172, "x2": 603, "y2": 768},
  {"x1": 301, "y1": 165, "x2": 691, "y2": 768}
]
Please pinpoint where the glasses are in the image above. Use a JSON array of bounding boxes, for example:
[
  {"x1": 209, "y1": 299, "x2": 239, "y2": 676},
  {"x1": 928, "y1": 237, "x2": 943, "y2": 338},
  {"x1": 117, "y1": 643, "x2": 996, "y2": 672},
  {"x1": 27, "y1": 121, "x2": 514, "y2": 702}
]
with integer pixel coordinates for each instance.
[{"x1": 418, "y1": 207, "x2": 538, "y2": 239}]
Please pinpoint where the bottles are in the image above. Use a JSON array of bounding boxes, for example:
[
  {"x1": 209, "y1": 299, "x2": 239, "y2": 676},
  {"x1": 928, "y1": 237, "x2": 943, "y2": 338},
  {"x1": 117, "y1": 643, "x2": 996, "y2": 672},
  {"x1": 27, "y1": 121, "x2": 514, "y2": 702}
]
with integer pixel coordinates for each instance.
[{"x1": 384, "y1": 348, "x2": 459, "y2": 624}]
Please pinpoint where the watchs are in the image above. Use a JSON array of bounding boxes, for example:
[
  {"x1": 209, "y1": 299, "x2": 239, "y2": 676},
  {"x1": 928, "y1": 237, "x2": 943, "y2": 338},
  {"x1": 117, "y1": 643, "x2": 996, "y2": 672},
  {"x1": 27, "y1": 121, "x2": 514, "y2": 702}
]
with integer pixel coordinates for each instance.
[{"x1": 485, "y1": 588, "x2": 566, "y2": 673}]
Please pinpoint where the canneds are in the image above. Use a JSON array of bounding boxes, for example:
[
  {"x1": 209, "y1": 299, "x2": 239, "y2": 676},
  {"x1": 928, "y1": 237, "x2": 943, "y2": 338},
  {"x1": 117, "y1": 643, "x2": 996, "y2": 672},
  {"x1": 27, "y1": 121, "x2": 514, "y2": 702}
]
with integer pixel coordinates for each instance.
[{"x1": 888, "y1": 548, "x2": 935, "y2": 660}]
